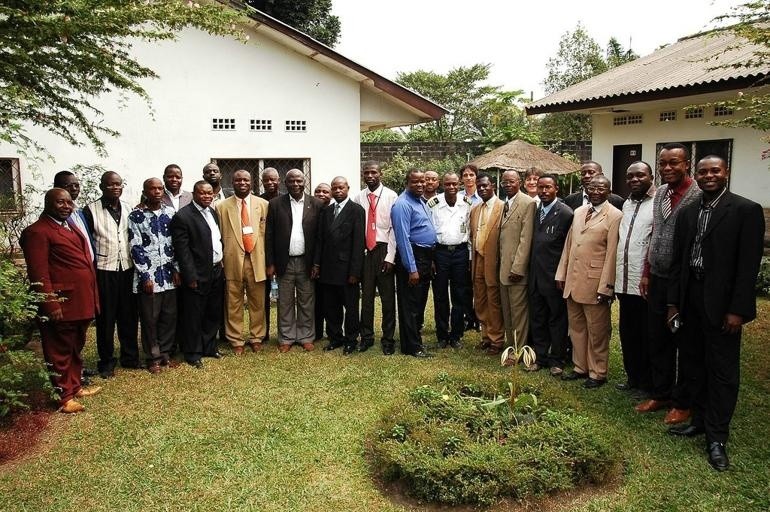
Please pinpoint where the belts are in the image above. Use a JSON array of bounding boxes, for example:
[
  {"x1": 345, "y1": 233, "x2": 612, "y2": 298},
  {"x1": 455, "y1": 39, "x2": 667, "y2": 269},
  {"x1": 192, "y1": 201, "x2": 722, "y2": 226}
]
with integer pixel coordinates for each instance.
[{"x1": 437, "y1": 244, "x2": 467, "y2": 251}]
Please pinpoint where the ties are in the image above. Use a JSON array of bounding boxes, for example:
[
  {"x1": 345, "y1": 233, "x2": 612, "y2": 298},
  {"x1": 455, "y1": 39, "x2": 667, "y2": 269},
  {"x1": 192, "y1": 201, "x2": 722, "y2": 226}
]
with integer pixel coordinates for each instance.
[
  {"x1": 202, "y1": 208, "x2": 221, "y2": 254},
  {"x1": 478, "y1": 204, "x2": 594, "y2": 257},
  {"x1": 661, "y1": 189, "x2": 674, "y2": 219},
  {"x1": 333, "y1": 204, "x2": 339, "y2": 218},
  {"x1": 366, "y1": 194, "x2": 376, "y2": 250},
  {"x1": 63, "y1": 211, "x2": 95, "y2": 263},
  {"x1": 241, "y1": 201, "x2": 255, "y2": 253}
]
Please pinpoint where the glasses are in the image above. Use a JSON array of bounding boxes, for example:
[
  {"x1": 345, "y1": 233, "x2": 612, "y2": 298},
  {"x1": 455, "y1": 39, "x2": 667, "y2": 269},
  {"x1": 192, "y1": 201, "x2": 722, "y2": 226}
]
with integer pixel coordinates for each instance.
[
  {"x1": 587, "y1": 186, "x2": 608, "y2": 194},
  {"x1": 658, "y1": 159, "x2": 687, "y2": 166}
]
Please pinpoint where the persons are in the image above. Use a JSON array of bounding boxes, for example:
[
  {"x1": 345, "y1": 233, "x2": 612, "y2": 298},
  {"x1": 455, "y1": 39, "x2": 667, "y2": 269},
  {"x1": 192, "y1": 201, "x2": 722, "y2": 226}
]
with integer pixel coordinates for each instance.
[
  {"x1": 18, "y1": 171, "x2": 146, "y2": 413},
  {"x1": 614, "y1": 160, "x2": 658, "y2": 401},
  {"x1": 634, "y1": 143, "x2": 705, "y2": 424},
  {"x1": 468, "y1": 162, "x2": 625, "y2": 390},
  {"x1": 128, "y1": 163, "x2": 482, "y2": 375},
  {"x1": 664, "y1": 155, "x2": 764, "y2": 472}
]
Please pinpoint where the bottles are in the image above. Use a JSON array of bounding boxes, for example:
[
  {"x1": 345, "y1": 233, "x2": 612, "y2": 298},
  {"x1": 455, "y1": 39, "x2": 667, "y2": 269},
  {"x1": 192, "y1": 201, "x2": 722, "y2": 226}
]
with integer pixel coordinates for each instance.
[{"x1": 270, "y1": 272, "x2": 279, "y2": 299}]
